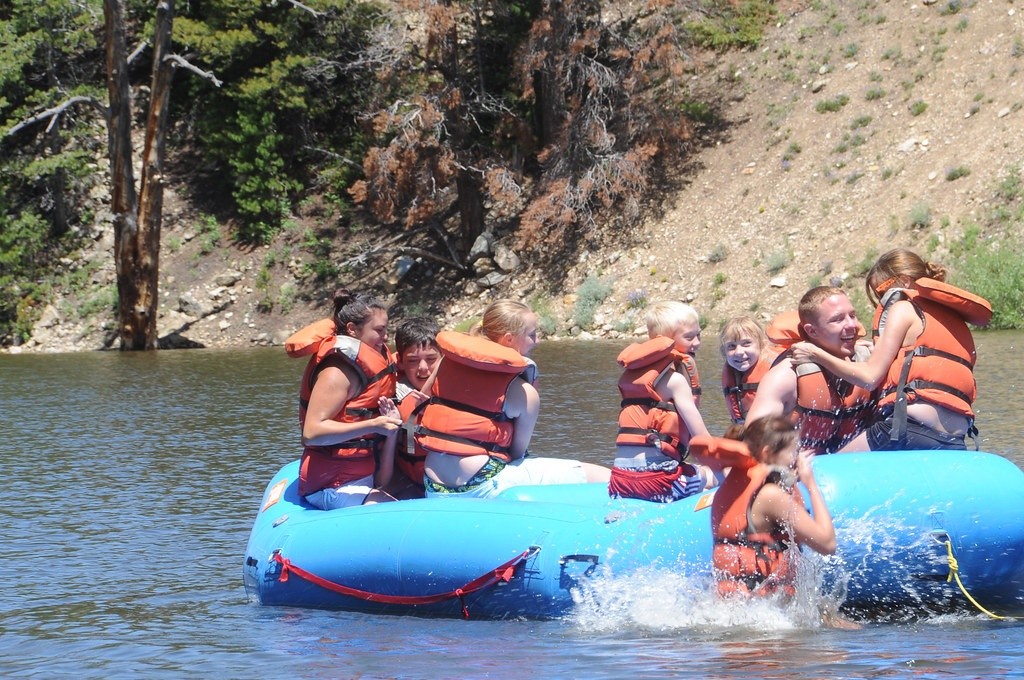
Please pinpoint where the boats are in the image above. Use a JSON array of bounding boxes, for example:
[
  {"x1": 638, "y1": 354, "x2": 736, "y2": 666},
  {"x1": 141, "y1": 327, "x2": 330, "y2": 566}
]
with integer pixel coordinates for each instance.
[{"x1": 244, "y1": 451, "x2": 1024, "y2": 623}]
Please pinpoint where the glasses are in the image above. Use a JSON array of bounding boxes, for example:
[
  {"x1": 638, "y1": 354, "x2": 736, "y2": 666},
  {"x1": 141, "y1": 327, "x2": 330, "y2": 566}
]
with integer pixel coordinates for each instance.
[{"x1": 875, "y1": 278, "x2": 899, "y2": 296}]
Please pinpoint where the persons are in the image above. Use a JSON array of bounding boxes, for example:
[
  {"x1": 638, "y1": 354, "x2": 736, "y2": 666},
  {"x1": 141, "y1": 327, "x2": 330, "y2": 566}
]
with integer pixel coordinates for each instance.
[
  {"x1": 299, "y1": 287, "x2": 613, "y2": 510},
  {"x1": 609, "y1": 251, "x2": 977, "y2": 503},
  {"x1": 712, "y1": 417, "x2": 861, "y2": 629}
]
